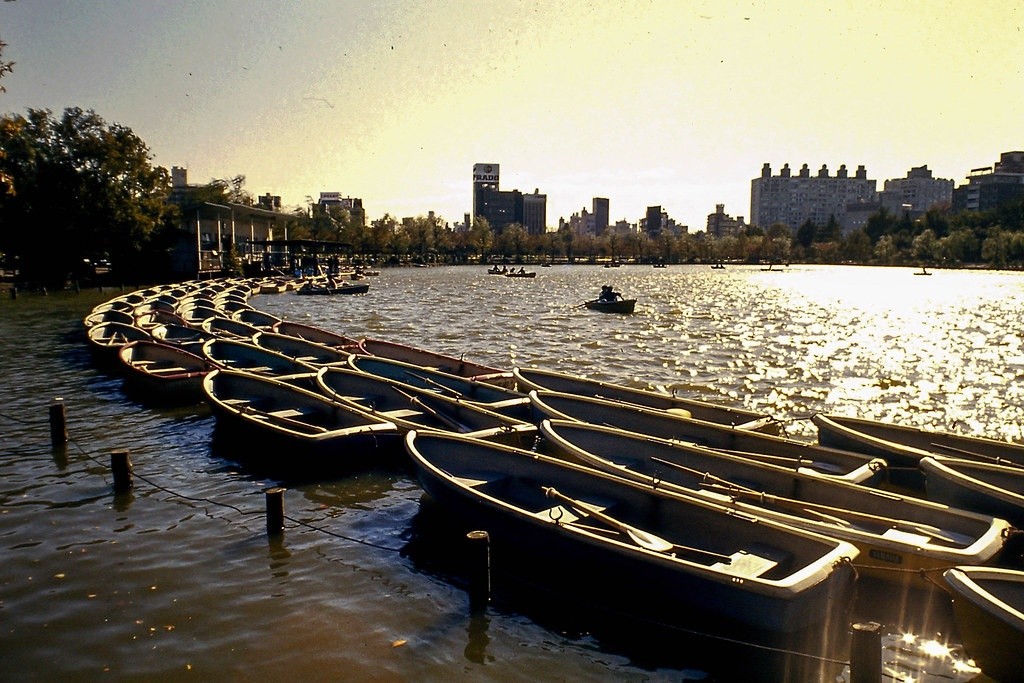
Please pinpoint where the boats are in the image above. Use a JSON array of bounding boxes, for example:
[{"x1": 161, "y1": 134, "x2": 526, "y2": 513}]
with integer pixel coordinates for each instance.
[
  {"x1": 488, "y1": 269, "x2": 508, "y2": 274},
  {"x1": 654, "y1": 265, "x2": 667, "y2": 268},
  {"x1": 940, "y1": 564, "x2": 1024, "y2": 683},
  {"x1": 918, "y1": 456, "x2": 1023, "y2": 532},
  {"x1": 201, "y1": 367, "x2": 398, "y2": 458},
  {"x1": 585, "y1": 298, "x2": 638, "y2": 314},
  {"x1": 505, "y1": 272, "x2": 536, "y2": 277},
  {"x1": 117, "y1": 339, "x2": 222, "y2": 392},
  {"x1": 538, "y1": 416, "x2": 1011, "y2": 599},
  {"x1": 526, "y1": 388, "x2": 889, "y2": 489},
  {"x1": 711, "y1": 266, "x2": 725, "y2": 269},
  {"x1": 511, "y1": 364, "x2": 783, "y2": 438},
  {"x1": 83, "y1": 263, "x2": 540, "y2": 455},
  {"x1": 315, "y1": 367, "x2": 538, "y2": 452},
  {"x1": 809, "y1": 412, "x2": 1024, "y2": 495},
  {"x1": 405, "y1": 427, "x2": 862, "y2": 633}
]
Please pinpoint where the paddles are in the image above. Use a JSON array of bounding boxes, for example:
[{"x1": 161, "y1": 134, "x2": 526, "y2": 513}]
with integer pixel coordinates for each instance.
[
  {"x1": 403, "y1": 369, "x2": 478, "y2": 401},
  {"x1": 930, "y1": 442, "x2": 1024, "y2": 470},
  {"x1": 391, "y1": 385, "x2": 474, "y2": 434},
  {"x1": 696, "y1": 480, "x2": 977, "y2": 547},
  {"x1": 107, "y1": 332, "x2": 129, "y2": 345},
  {"x1": 649, "y1": 454, "x2": 870, "y2": 531},
  {"x1": 561, "y1": 520, "x2": 734, "y2": 564},
  {"x1": 699, "y1": 445, "x2": 849, "y2": 475},
  {"x1": 231, "y1": 404, "x2": 326, "y2": 433},
  {"x1": 540, "y1": 484, "x2": 674, "y2": 553}
]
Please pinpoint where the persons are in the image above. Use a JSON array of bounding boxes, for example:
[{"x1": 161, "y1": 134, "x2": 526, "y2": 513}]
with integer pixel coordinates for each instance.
[
  {"x1": 502, "y1": 266, "x2": 508, "y2": 272},
  {"x1": 598, "y1": 285, "x2": 622, "y2": 302},
  {"x1": 493, "y1": 265, "x2": 500, "y2": 272},
  {"x1": 605, "y1": 261, "x2": 608, "y2": 266},
  {"x1": 326, "y1": 275, "x2": 337, "y2": 289},
  {"x1": 612, "y1": 259, "x2": 615, "y2": 265},
  {"x1": 716, "y1": 262, "x2": 723, "y2": 267},
  {"x1": 518, "y1": 267, "x2": 525, "y2": 274},
  {"x1": 658, "y1": 263, "x2": 664, "y2": 266},
  {"x1": 510, "y1": 267, "x2": 515, "y2": 273}
]
